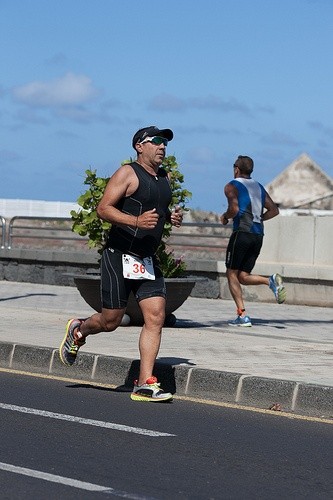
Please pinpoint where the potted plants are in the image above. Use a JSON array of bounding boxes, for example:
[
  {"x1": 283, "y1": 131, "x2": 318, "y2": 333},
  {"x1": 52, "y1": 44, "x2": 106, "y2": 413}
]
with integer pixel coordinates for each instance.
[{"x1": 61, "y1": 154, "x2": 209, "y2": 325}]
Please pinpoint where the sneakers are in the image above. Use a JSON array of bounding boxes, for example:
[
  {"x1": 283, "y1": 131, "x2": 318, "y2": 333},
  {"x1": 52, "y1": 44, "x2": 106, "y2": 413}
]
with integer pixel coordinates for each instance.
[
  {"x1": 228, "y1": 314, "x2": 252, "y2": 327},
  {"x1": 59, "y1": 319, "x2": 86, "y2": 366},
  {"x1": 268, "y1": 274, "x2": 286, "y2": 304},
  {"x1": 130, "y1": 377, "x2": 173, "y2": 402}
]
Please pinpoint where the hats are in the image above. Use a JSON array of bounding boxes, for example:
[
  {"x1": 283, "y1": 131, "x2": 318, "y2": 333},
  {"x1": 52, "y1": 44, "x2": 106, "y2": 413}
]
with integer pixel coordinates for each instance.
[{"x1": 132, "y1": 126, "x2": 174, "y2": 147}]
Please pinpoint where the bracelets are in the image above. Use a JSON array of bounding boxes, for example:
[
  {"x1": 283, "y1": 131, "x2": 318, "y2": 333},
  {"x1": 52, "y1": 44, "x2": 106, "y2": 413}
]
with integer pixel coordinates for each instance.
[
  {"x1": 223, "y1": 212, "x2": 228, "y2": 220},
  {"x1": 135, "y1": 216, "x2": 138, "y2": 227}
]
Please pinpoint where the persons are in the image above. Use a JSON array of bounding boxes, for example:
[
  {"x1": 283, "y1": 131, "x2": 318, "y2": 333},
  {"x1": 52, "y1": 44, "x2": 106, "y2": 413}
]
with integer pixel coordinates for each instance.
[
  {"x1": 59, "y1": 126, "x2": 185, "y2": 403},
  {"x1": 221, "y1": 156, "x2": 287, "y2": 328}
]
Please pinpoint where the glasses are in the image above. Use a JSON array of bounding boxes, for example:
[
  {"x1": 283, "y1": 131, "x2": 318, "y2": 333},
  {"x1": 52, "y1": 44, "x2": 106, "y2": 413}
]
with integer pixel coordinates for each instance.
[
  {"x1": 139, "y1": 136, "x2": 168, "y2": 146},
  {"x1": 233, "y1": 164, "x2": 239, "y2": 168}
]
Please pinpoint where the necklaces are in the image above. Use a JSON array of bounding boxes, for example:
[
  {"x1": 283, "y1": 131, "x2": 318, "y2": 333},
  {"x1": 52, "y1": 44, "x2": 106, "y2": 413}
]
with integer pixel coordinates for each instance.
[{"x1": 139, "y1": 161, "x2": 159, "y2": 181}]
